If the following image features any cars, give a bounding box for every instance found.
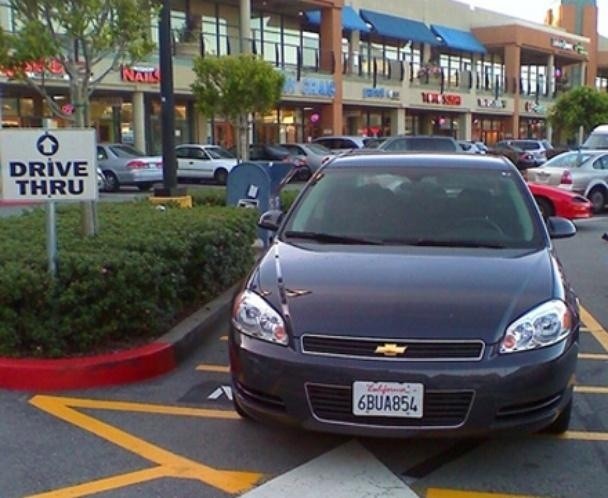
[524,124,608,216]
[314,135,490,154]
[175,144,241,183]
[226,138,336,179]
[225,149,582,442]
[95,141,163,191]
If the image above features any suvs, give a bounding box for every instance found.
[494,139,554,169]
